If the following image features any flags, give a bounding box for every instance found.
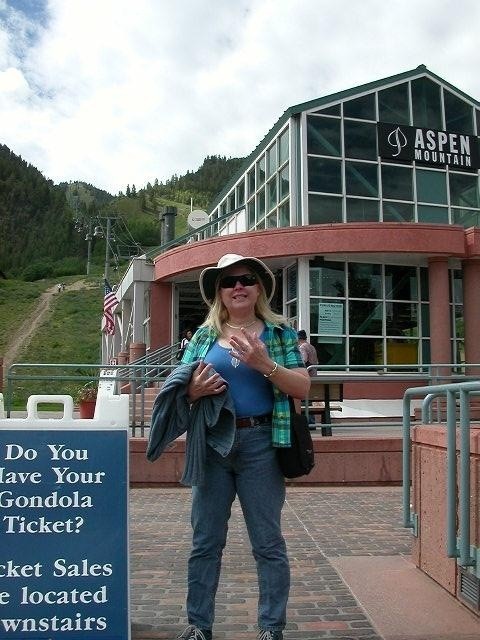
[101,278,119,335]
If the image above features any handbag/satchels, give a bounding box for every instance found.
[275,394,316,480]
[176,338,186,359]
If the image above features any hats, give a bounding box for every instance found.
[198,253,277,309]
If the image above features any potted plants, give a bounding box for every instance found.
[67,383,97,419]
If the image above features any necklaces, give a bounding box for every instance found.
[225,318,258,330]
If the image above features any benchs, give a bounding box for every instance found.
[297,405,342,437]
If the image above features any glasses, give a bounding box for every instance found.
[217,273,260,290]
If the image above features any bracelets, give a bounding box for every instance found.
[264,362,278,378]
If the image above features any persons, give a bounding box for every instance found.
[146,253,311,640]
[181,329,193,349]
[297,330,318,430]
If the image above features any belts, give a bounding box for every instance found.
[234,411,272,429]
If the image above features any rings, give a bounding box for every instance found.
[241,351,243,354]
